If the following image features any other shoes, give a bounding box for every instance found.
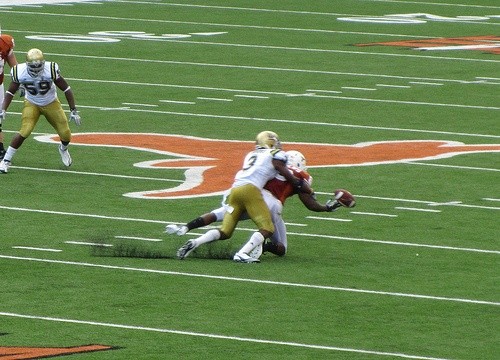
[0,150,6,159]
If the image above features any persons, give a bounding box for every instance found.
[165,149,343,260]
[177,130,313,263]
[0,48,81,173]
[0,34,19,159]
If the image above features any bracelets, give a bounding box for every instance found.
[71,108,75,110]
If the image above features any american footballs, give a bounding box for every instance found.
[335,188,357,209]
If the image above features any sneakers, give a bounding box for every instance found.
[59,144,72,167]
[0,158,11,173]
[250,238,266,259]
[176,239,198,260]
[166,225,189,236]
[233,252,261,263]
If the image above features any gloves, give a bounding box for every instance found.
[0,107,6,120]
[70,108,81,126]
[326,199,343,212]
[17,83,25,99]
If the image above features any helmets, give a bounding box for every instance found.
[255,131,280,149]
[286,150,306,170]
[26,48,45,67]
